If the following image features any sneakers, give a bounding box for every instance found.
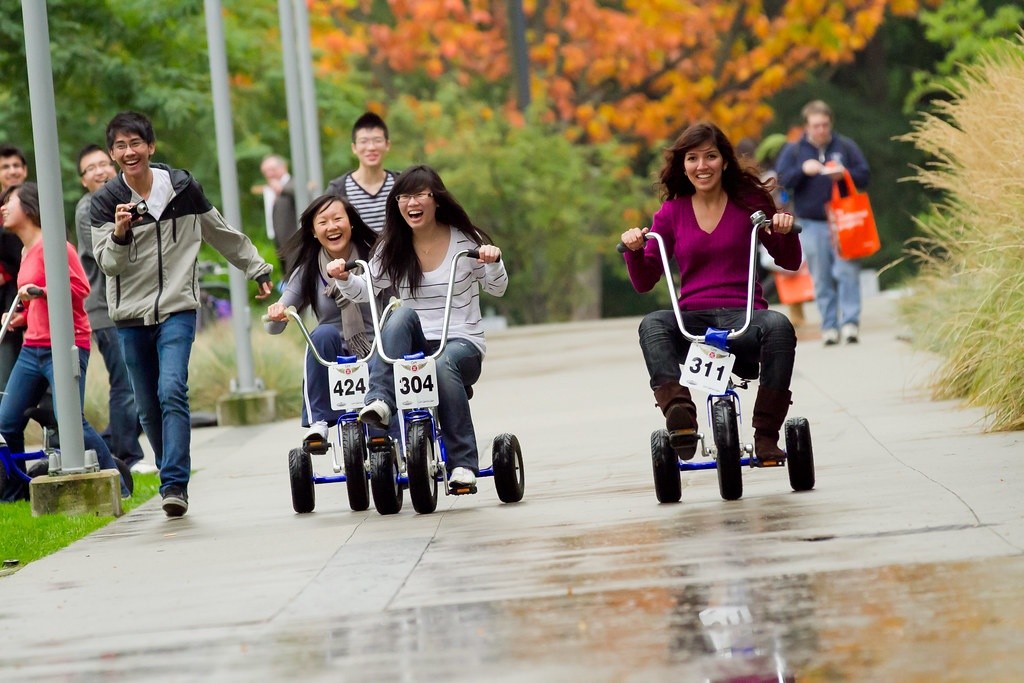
[448,466,477,489]
[162,484,188,516]
[360,400,390,431]
[303,421,329,455]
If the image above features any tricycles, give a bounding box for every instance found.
[615,208,817,502]
[0,286,137,502]
[261,297,413,513]
[327,249,525,514]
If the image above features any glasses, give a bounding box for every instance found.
[394,192,434,202]
[80,160,113,176]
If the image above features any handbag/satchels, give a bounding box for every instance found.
[823,162,881,260]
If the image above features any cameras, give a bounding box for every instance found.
[126,199,149,223]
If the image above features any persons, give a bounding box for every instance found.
[76,145,144,470]
[0,182,131,501]
[265,193,400,475]
[327,165,508,488]
[325,111,401,234]
[738,99,871,342]
[0,146,60,478]
[91,112,273,520]
[261,156,296,275]
[623,123,805,461]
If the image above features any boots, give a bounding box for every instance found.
[654,380,698,460]
[752,388,792,462]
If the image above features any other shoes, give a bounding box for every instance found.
[129,458,158,476]
[841,322,859,343]
[822,326,838,346]
